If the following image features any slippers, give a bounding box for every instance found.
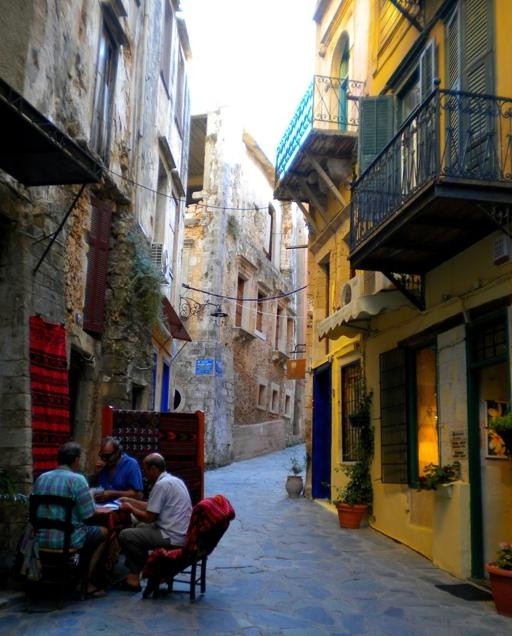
[86,588,106,597]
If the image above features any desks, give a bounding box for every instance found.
[83,498,133,562]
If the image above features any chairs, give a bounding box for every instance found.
[24,493,91,610]
[152,496,223,600]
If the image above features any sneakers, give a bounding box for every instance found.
[114,580,141,591]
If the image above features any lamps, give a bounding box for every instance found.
[179,296,228,327]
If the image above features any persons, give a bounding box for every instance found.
[31,439,192,598]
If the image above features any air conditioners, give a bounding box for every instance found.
[149,242,169,276]
[340,277,361,308]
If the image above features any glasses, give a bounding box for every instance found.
[98,451,115,458]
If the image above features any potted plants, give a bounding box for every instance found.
[286,456,307,498]
[333,377,375,529]
[485,542,511,618]
